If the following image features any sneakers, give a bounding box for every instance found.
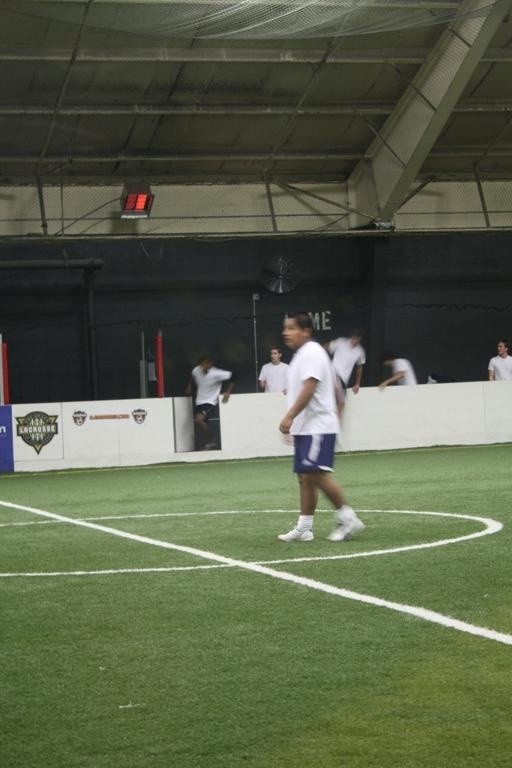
[204,442,218,449]
[327,519,366,542]
[278,527,314,543]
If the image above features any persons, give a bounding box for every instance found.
[487,337,512,381]
[278,311,365,543]
[323,327,366,393]
[257,348,288,393]
[377,350,418,390]
[186,355,234,450]
